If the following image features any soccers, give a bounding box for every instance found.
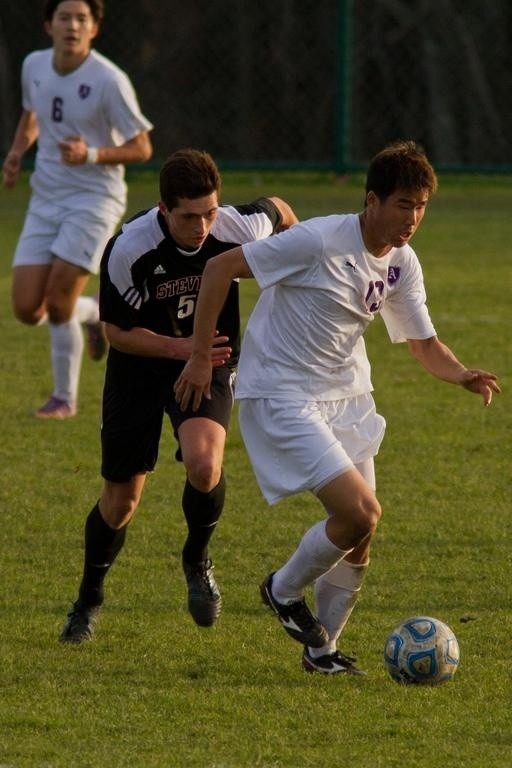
[384,617,459,684]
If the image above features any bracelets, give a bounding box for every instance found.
[83,148,98,167]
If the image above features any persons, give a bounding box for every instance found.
[173,142,502,677]
[59,147,300,644]
[3,0,156,419]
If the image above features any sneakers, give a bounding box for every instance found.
[34,396,76,418]
[260,572,364,676]
[59,603,101,642]
[183,559,221,627]
[87,295,108,360]
